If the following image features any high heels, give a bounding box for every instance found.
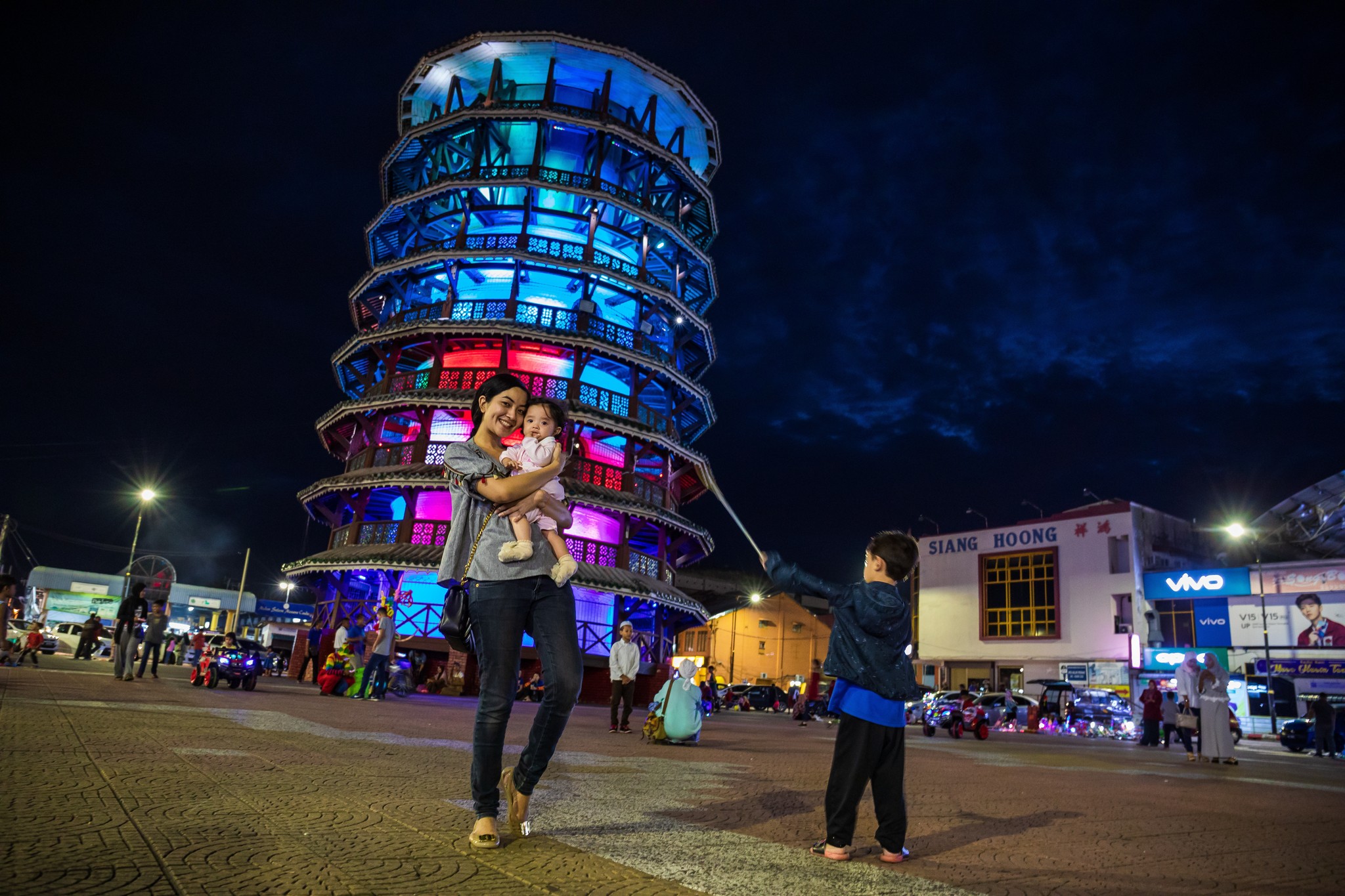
[1187,752,1195,761]
[1197,752,1208,763]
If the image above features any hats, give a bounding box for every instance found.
[620,621,632,629]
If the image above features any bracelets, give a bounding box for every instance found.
[541,496,550,510]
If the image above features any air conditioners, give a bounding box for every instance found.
[1118,623,1133,633]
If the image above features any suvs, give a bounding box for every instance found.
[1278,703,1345,753]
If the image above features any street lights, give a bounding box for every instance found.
[729,594,760,684]
[279,582,295,604]
[1226,523,1277,735]
[121,488,155,598]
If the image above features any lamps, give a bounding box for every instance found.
[1021,499,1043,519]
[918,514,939,535]
[1084,488,1101,502]
[966,508,988,529]
[577,299,596,313]
[637,320,653,335]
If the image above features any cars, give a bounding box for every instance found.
[131,628,288,673]
[716,683,787,713]
[1227,707,1242,746]
[907,690,980,721]
[921,698,989,741]
[50,622,113,659]
[3,618,60,655]
[191,645,257,692]
[971,692,1039,727]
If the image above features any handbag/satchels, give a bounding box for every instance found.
[438,585,474,654]
[641,712,667,744]
[1176,708,1197,729]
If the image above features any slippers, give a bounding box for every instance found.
[881,847,910,863]
[810,839,850,861]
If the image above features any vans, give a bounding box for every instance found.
[1025,679,1133,729]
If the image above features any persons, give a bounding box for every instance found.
[649,658,704,744]
[15,622,45,668]
[765,683,779,714]
[84,616,103,659]
[498,398,578,588]
[408,648,427,685]
[959,682,976,713]
[188,628,205,667]
[1004,690,1018,721]
[262,645,276,677]
[797,658,838,729]
[0,571,18,651]
[114,581,148,681]
[515,669,544,702]
[317,606,398,701]
[1174,650,1210,762]
[1295,593,1345,646]
[296,619,324,685]
[1300,692,1337,758]
[439,661,465,696]
[134,599,168,679]
[219,632,237,656]
[72,613,100,660]
[792,694,816,722]
[175,632,190,666]
[762,530,919,861]
[159,629,177,665]
[426,665,448,694]
[274,651,284,677]
[1136,679,1189,750]
[609,621,640,733]
[1195,652,1239,766]
[699,665,751,717]
[435,372,582,849]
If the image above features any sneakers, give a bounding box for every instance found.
[349,693,365,701]
[366,693,381,702]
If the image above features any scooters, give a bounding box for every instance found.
[387,659,414,699]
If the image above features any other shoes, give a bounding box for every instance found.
[1223,758,1239,766]
[798,722,807,727]
[124,674,134,680]
[502,766,530,838]
[116,676,122,681]
[620,725,632,733]
[469,818,500,848]
[1212,757,1219,764]
[609,725,618,733]
